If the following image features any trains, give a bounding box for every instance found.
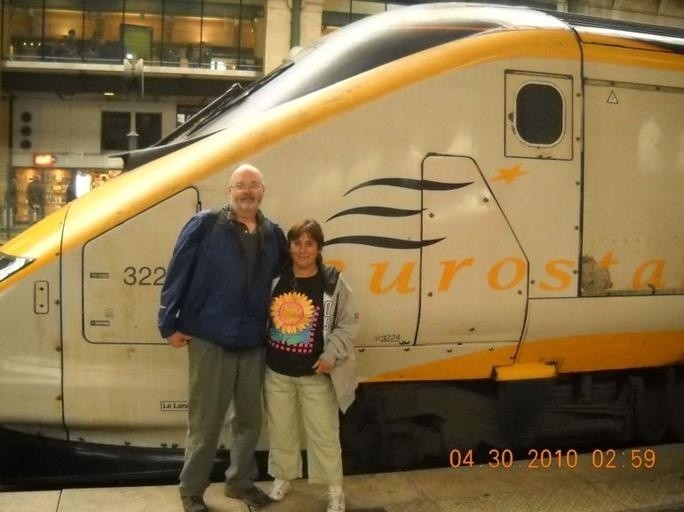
[0,1,684,464]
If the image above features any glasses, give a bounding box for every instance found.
[229,182,262,191]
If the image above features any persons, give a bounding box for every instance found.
[5,175,45,226]
[167,41,212,69]
[64,29,107,58]
[158,163,289,512]
[262,219,359,512]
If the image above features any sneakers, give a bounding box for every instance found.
[180,495,209,512]
[267,477,293,503]
[326,484,346,512]
[224,487,272,508]
